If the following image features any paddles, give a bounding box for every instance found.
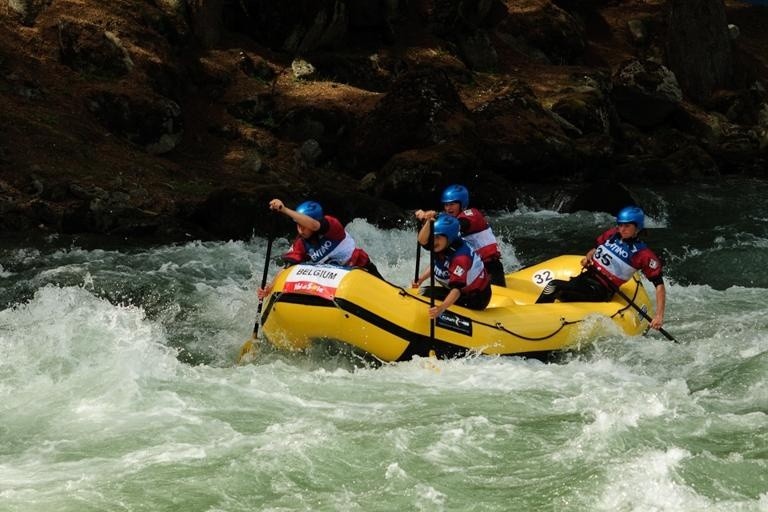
[234,201,282,366]
[419,213,444,375]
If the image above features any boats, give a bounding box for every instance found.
[260,253,654,365]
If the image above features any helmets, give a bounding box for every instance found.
[615,205,645,232]
[432,212,463,246]
[441,183,470,211]
[295,200,324,221]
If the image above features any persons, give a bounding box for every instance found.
[258,199,384,300]
[417,211,491,320]
[411,185,507,289]
[535,207,665,329]
